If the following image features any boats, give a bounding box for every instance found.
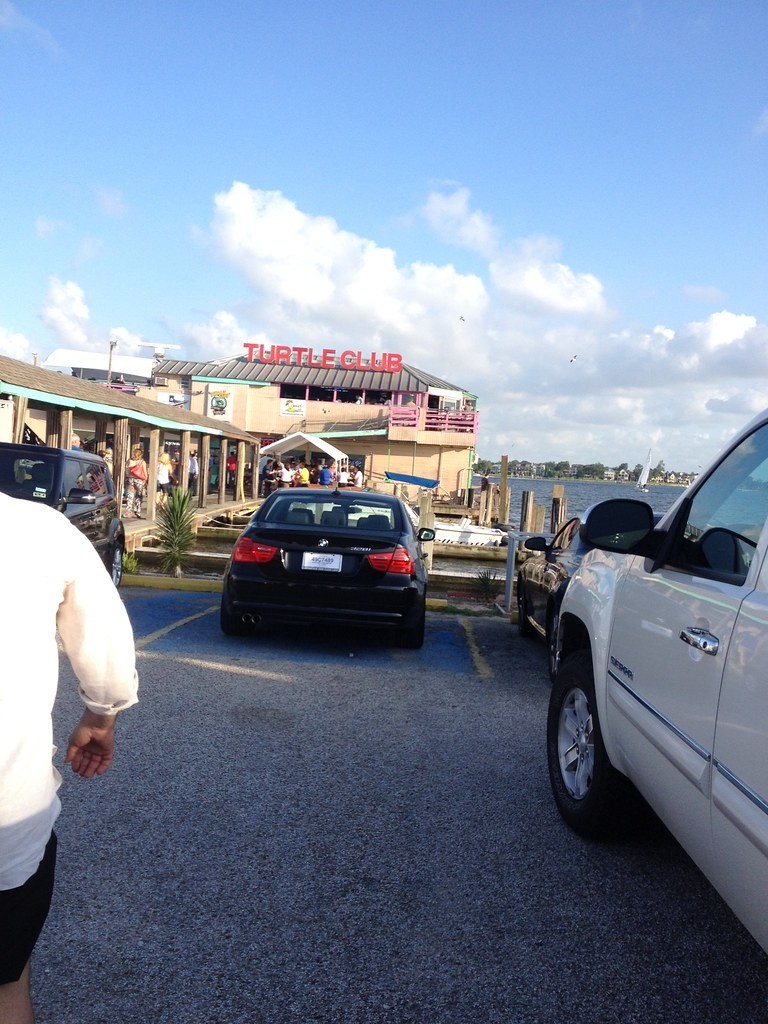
[384,472,514,547]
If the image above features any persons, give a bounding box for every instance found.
[262,459,363,489]
[112,377,125,384]
[482,473,490,491]
[72,433,86,451]
[157,452,178,506]
[464,401,474,410]
[384,396,393,405]
[354,394,365,405]
[96,449,114,478]
[244,464,252,485]
[226,452,237,488]
[0,490,140,1023]
[122,448,147,518]
[407,398,416,405]
[188,454,199,496]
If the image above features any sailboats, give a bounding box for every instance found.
[635,448,652,493]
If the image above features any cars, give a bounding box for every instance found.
[515,512,672,681]
[220,487,436,652]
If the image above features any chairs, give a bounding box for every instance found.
[21,464,53,492]
[285,509,390,532]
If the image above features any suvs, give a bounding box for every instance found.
[0,441,127,591]
[540,405,768,958]
